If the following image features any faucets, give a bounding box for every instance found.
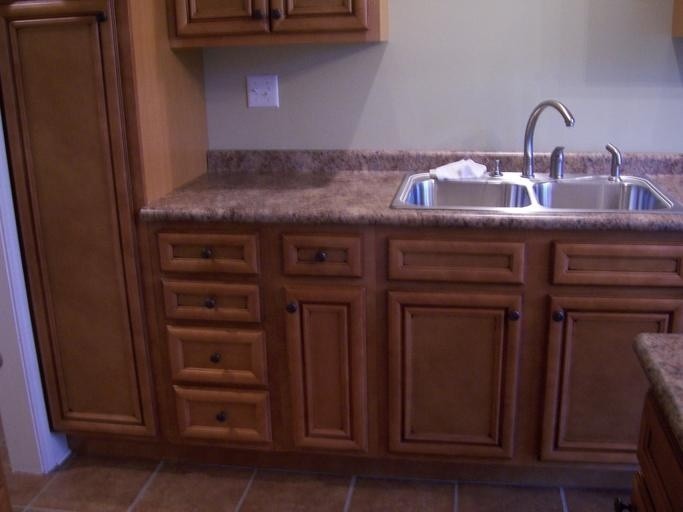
[523,100,575,177]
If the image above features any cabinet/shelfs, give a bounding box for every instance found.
[0,2,208,463]
[541,227,683,489]
[383,223,528,492]
[164,1,389,49]
[139,223,278,491]
[282,224,372,491]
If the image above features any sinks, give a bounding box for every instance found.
[533,175,683,214]
[390,172,533,215]
